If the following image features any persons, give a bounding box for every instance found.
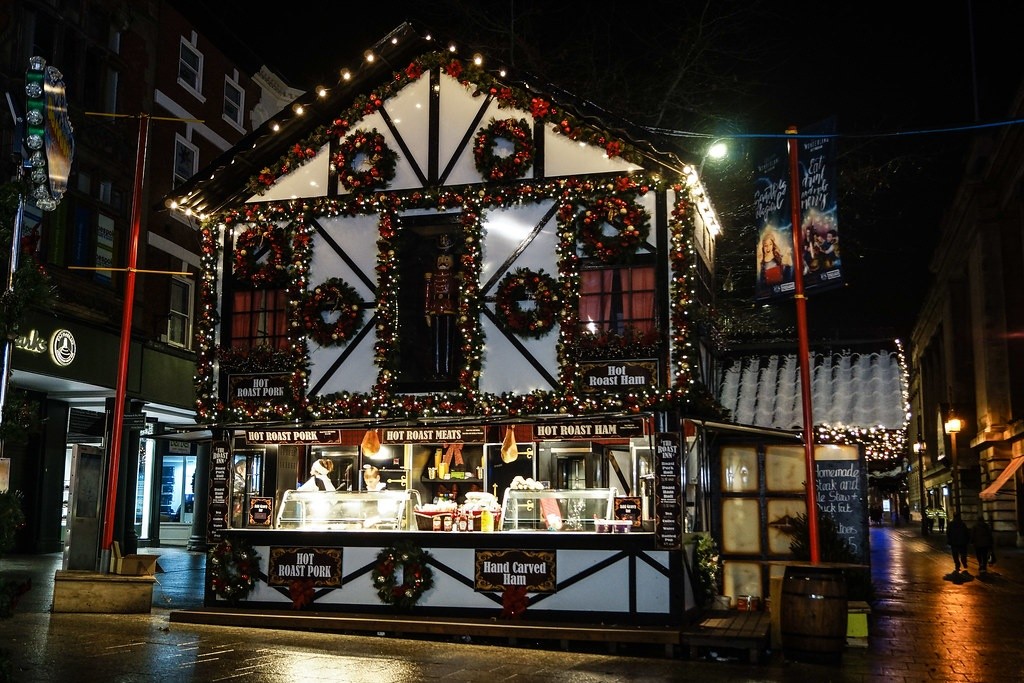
[760,234,793,287]
[425,233,460,381]
[869,504,883,524]
[973,514,993,571]
[800,208,840,273]
[948,513,969,570]
[363,466,388,494]
[233,460,246,493]
[297,459,337,492]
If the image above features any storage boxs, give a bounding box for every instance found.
[113,541,165,576]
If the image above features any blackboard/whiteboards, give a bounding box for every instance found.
[812,457,871,599]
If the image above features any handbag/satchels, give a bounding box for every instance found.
[987,552,996,564]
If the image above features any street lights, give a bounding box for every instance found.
[913,433,930,537]
[944,418,962,519]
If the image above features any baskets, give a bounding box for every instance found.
[413,510,501,531]
